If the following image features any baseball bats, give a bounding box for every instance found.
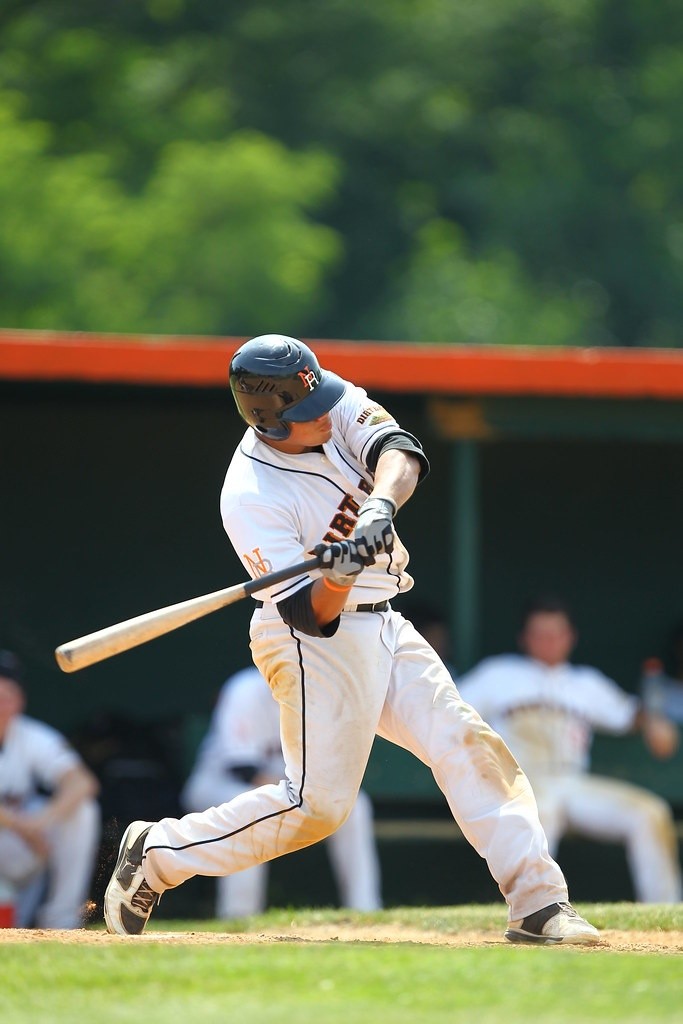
[53,557,321,677]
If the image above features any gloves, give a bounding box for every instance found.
[314,539,365,591]
[350,495,398,567]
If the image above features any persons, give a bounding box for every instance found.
[1,667,100,928]
[105,334,600,944]
[186,667,382,917]
[456,609,681,902]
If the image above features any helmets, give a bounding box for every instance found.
[228,334,346,443]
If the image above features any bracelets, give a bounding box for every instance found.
[324,577,352,592]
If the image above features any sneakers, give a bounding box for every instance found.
[504,900,599,946]
[103,820,160,936]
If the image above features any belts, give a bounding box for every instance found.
[255,600,391,614]
